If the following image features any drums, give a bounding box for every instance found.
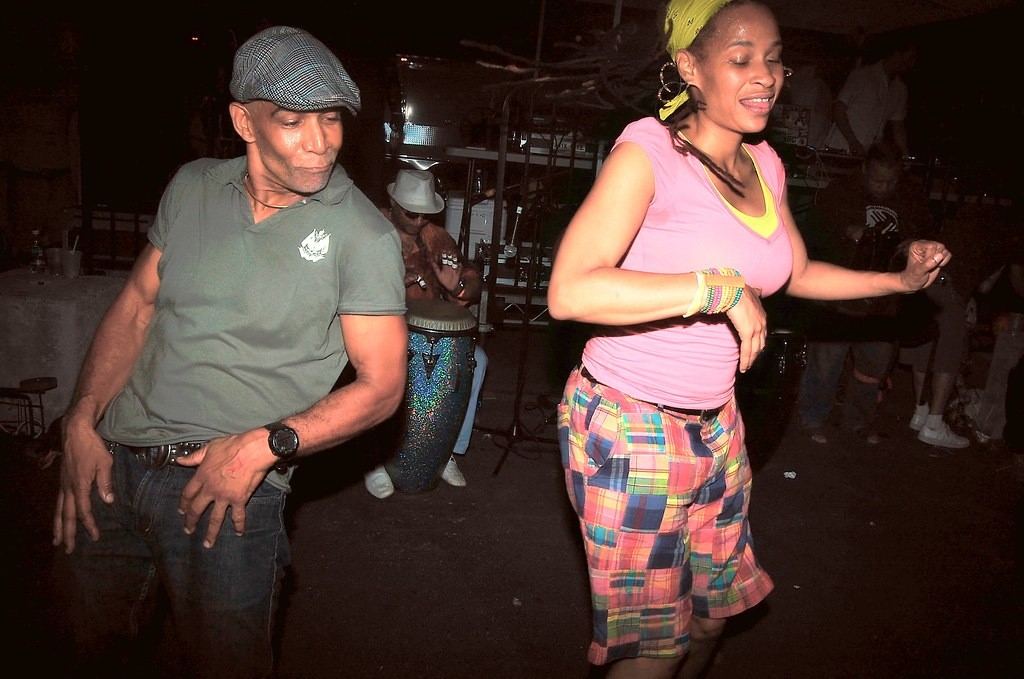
[379,297,479,494]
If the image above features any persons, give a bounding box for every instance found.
[800,143,1024,448]
[51,27,407,679]
[547,0,953,679]
[825,35,917,161]
[365,169,489,498]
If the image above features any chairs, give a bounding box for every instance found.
[815,236,942,444]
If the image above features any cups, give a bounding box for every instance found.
[45,248,67,276]
[62,250,82,278]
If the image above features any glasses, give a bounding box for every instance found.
[402,208,430,219]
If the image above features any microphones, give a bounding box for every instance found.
[504,200,525,257]
[542,80,596,103]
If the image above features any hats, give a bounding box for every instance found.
[387,168,445,213]
[230,26,361,117]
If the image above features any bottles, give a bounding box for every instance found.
[29,230,45,280]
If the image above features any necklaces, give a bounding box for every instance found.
[245,173,287,208]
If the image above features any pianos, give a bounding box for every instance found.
[805,145,941,173]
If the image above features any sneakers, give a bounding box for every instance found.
[917,422,970,449]
[909,414,930,431]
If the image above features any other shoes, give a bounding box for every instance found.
[808,429,833,448]
[361,461,393,498]
[442,456,467,488]
[855,429,881,446]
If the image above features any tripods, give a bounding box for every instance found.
[473,102,559,478]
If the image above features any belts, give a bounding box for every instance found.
[131,441,209,470]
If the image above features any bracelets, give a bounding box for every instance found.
[450,280,464,298]
[683,267,746,318]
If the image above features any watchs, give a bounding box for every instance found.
[263,421,299,470]
